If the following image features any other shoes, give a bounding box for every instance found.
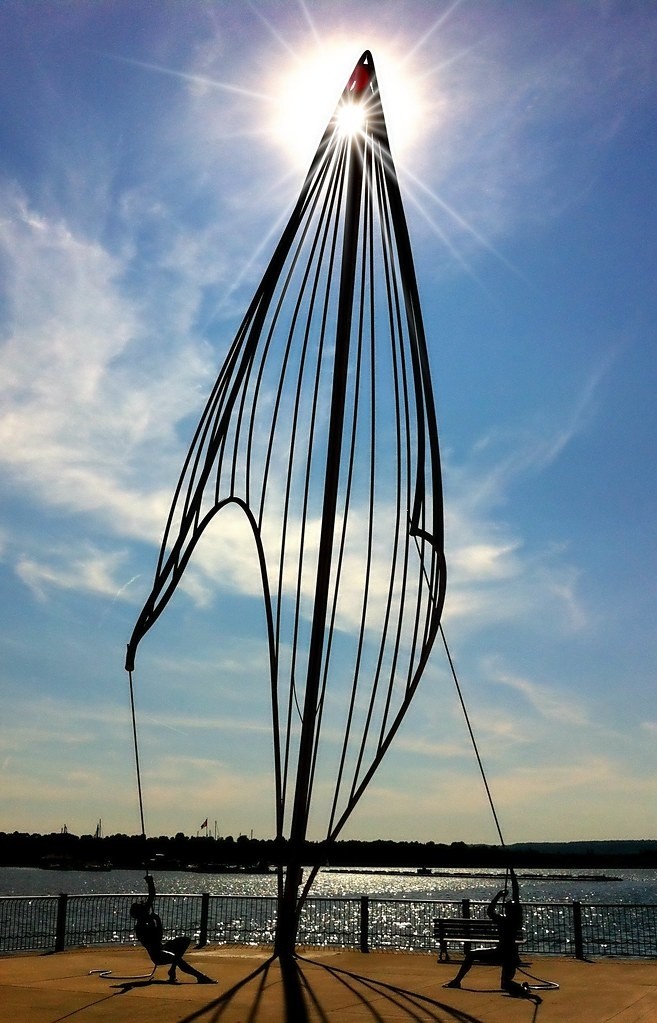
[197,975,219,984]
[168,971,178,981]
[442,980,461,988]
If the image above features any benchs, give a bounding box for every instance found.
[434,918,528,963]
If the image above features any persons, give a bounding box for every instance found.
[443,863,530,993]
[130,874,220,984]
